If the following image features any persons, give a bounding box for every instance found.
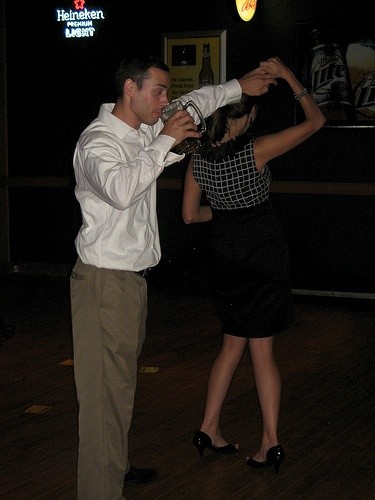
[182,57,326,467]
[70,54,276,500]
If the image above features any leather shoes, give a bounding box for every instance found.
[123,466,159,485]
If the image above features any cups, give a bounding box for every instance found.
[157,101,207,155]
[344,43,375,121]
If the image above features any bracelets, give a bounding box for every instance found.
[294,88,308,100]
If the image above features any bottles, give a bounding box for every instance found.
[198,42,214,89]
[307,26,356,125]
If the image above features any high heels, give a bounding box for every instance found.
[192,428,239,463]
[246,444,286,474]
